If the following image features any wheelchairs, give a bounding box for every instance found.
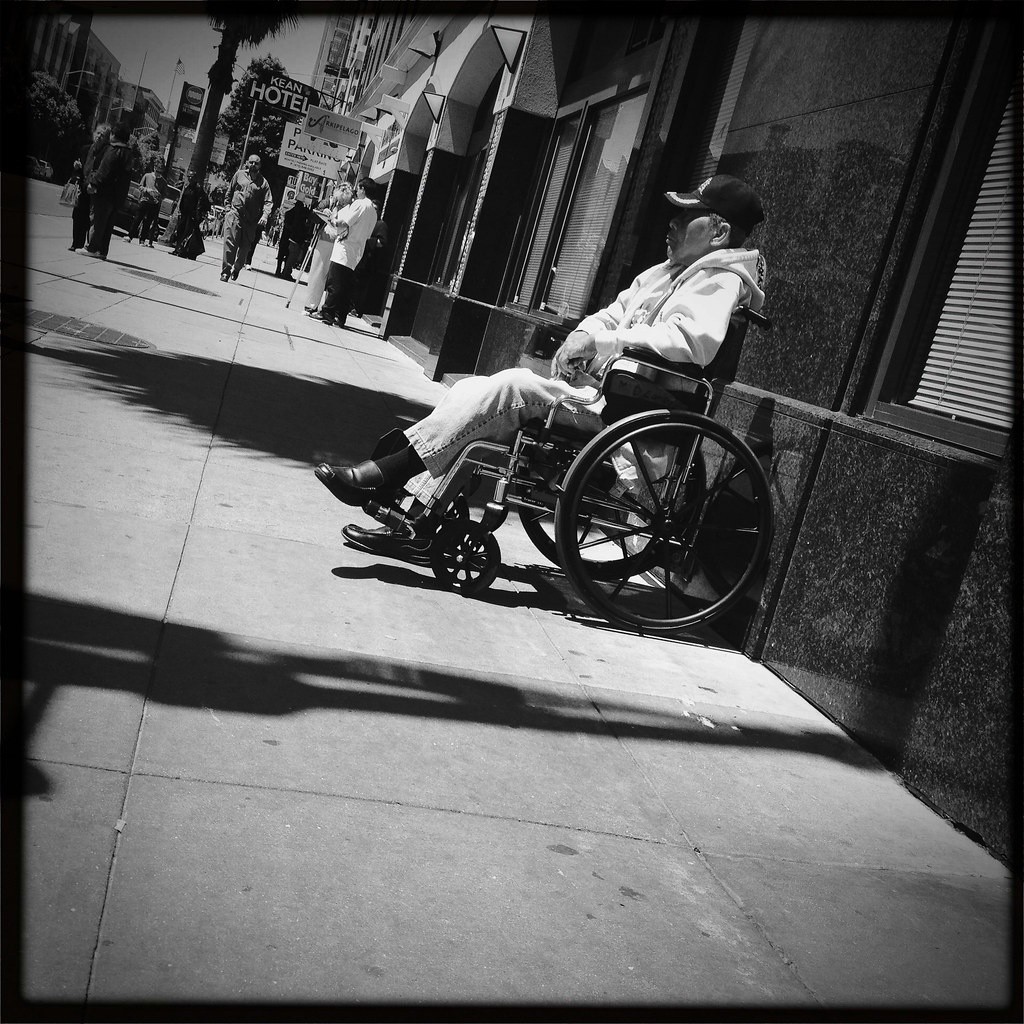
[357,297,780,639]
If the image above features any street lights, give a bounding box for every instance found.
[132,126,155,137]
[59,71,95,93]
[106,107,133,124]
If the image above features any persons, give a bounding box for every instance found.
[67,122,133,261]
[300,177,389,329]
[266,226,280,246]
[219,154,273,280]
[124,158,167,249]
[167,170,209,258]
[312,173,766,570]
[274,200,323,282]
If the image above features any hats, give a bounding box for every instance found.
[663,174,765,237]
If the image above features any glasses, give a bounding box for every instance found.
[155,170,162,174]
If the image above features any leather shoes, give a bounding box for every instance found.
[316,459,391,506]
[340,524,433,567]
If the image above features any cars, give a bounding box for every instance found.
[113,181,160,243]
[154,184,183,235]
[27,155,54,180]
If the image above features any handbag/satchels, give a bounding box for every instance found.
[58,169,82,208]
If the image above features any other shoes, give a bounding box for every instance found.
[68,247,78,251]
[220,273,229,281]
[332,321,343,328]
[139,242,145,247]
[303,306,317,317]
[76,248,106,259]
[168,250,187,258]
[308,312,331,327]
[231,271,239,280]
[275,272,293,281]
[244,264,251,271]
[122,236,131,243]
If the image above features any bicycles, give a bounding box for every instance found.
[203,205,224,241]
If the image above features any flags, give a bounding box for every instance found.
[176,59,185,77]
